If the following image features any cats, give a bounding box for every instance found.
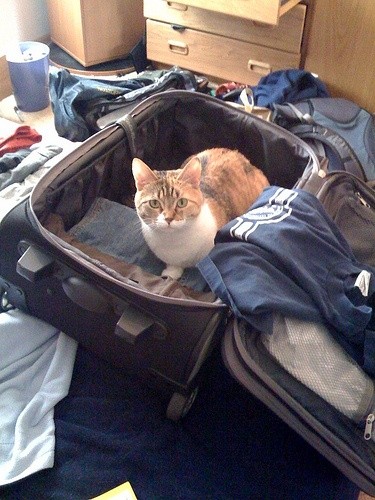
[130,147,271,280]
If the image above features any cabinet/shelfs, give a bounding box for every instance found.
[46,0,146,67]
[142,0,375,114]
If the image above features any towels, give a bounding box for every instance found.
[0,308,77,486]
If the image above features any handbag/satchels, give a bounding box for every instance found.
[225,88,273,123]
[48,65,197,142]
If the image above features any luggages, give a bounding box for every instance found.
[0,90,375,499]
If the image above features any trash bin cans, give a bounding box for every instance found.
[5,40,51,112]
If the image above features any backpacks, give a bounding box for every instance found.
[272,97,375,190]
